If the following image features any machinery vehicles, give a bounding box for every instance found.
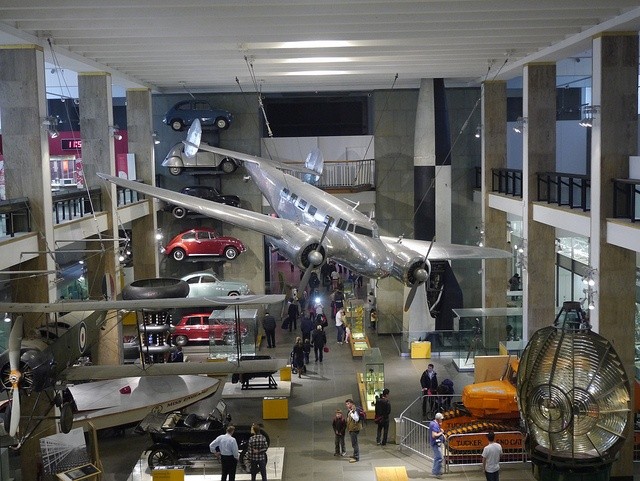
[439,354,591,461]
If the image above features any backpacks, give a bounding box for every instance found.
[356,407,366,423]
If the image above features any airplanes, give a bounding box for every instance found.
[0,272,290,449]
[95,118,512,311]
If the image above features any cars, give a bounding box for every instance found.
[173,314,248,345]
[163,187,241,220]
[181,272,249,297]
[163,141,241,176]
[165,228,245,261]
[162,100,232,131]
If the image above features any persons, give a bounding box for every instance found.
[344,322,352,345]
[301,260,374,324]
[287,299,300,332]
[428,412,447,480]
[332,410,346,458]
[247,425,269,481]
[335,307,346,345]
[303,339,312,365]
[420,364,438,424]
[370,309,376,331]
[261,312,277,349]
[509,274,521,302]
[373,389,391,446]
[481,431,504,481]
[292,337,307,379]
[345,399,364,464]
[435,379,456,415]
[299,314,313,343]
[208,426,240,481]
[311,325,327,364]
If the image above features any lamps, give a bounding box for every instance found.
[580,267,596,286]
[118,245,130,263]
[577,103,598,129]
[515,244,527,253]
[511,115,527,133]
[43,115,59,138]
[151,130,161,145]
[78,257,85,266]
[112,123,123,141]
[474,125,483,139]
[155,227,163,242]
[581,288,597,308]
[3,311,11,323]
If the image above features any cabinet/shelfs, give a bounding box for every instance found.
[341,279,370,357]
[207,308,258,376]
[357,348,384,420]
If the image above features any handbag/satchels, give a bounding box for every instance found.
[323,347,329,353]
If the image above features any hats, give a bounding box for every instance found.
[435,413,444,419]
